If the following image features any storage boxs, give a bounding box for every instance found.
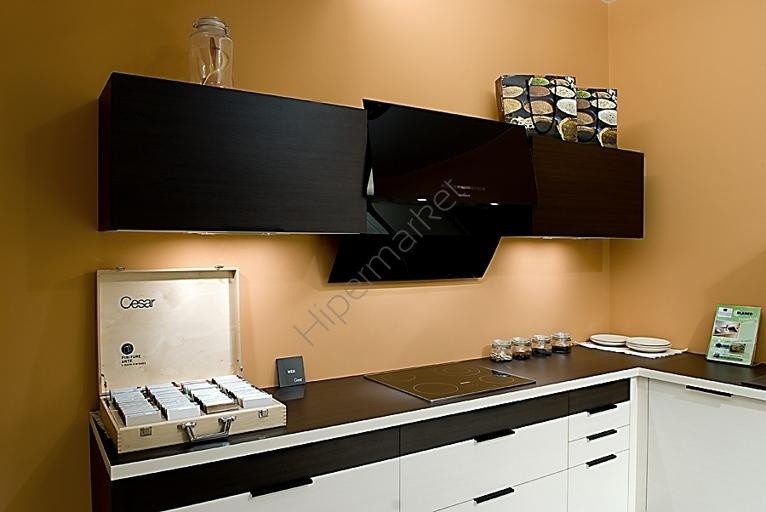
[91,263,288,454]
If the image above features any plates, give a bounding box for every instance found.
[589,334,628,346]
[625,337,672,353]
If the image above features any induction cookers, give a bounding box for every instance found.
[365,362,535,403]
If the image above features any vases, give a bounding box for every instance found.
[188,11,237,90]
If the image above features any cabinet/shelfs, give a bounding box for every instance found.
[83,339,642,512]
[633,350,766,512]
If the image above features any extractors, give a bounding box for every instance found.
[328,99,539,282]
